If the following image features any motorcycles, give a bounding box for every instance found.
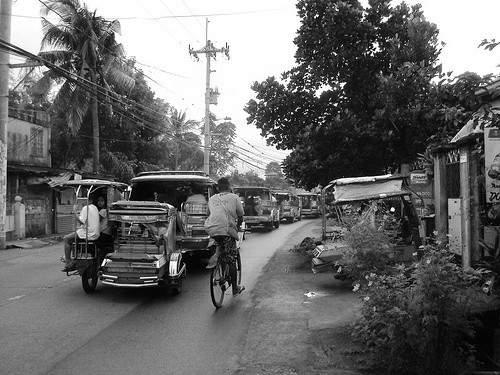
[65,179,128,294]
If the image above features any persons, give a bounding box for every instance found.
[60,193,101,272]
[203,177,245,297]
[60,195,114,263]
[176,183,208,237]
[254,191,268,216]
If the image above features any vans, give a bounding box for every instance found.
[297,193,323,217]
[227,187,279,232]
[273,193,301,223]
[131,171,245,266]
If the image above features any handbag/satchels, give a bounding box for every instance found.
[228,221,239,240]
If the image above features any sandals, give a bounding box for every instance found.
[233,286,245,297]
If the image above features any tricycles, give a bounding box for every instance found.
[98,200,186,295]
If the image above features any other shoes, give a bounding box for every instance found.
[61,266,71,272]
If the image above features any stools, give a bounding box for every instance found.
[73,241,97,258]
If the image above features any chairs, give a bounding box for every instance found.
[185,194,207,234]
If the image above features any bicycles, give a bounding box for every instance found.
[209,228,251,308]
